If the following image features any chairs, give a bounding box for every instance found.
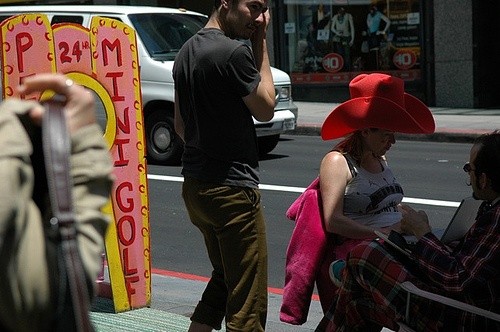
[397,281,500,332]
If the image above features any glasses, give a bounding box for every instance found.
[464,163,480,174]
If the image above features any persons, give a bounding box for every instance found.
[172,0,275,332]
[330,8,355,72]
[366,6,391,69]
[315,72,436,315]
[313,128,500,332]
[0,73,117,332]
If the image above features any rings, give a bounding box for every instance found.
[59,77,74,96]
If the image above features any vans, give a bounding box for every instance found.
[0,4,299,166]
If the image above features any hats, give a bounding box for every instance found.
[320,73,435,139]
